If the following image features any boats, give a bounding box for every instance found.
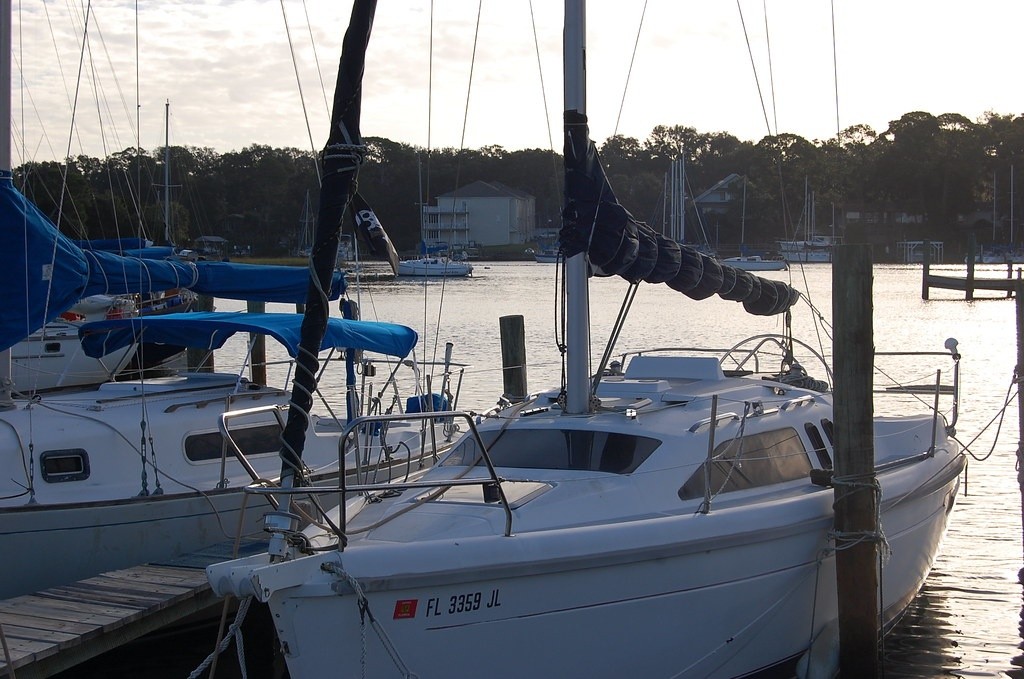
[0,0,974,679]
